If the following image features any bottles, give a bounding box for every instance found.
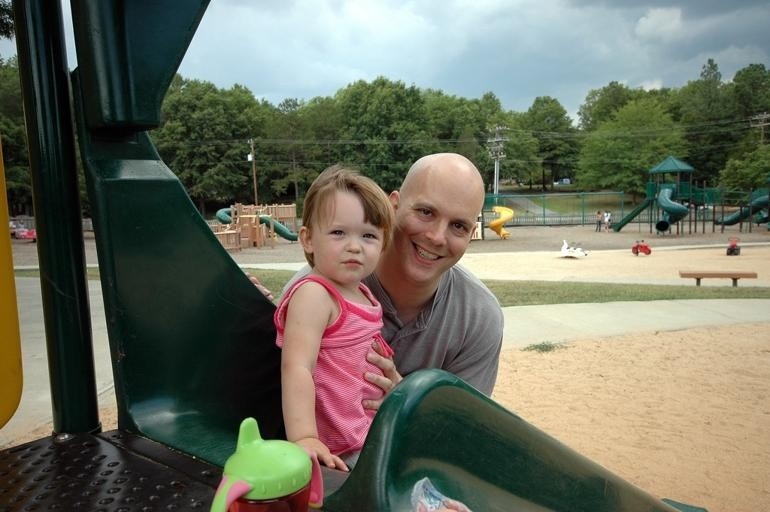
[210,416,325,512]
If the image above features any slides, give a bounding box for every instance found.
[655,188,688,231]
[715,196,770,225]
[79,124,706,512]
[490,206,514,240]
[607,182,655,232]
[217,209,298,241]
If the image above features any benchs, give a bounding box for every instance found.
[679,268,759,287]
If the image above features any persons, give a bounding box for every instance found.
[416,495,471,512]
[604,211,612,230]
[272,164,397,475]
[595,211,602,232]
[244,152,502,419]
